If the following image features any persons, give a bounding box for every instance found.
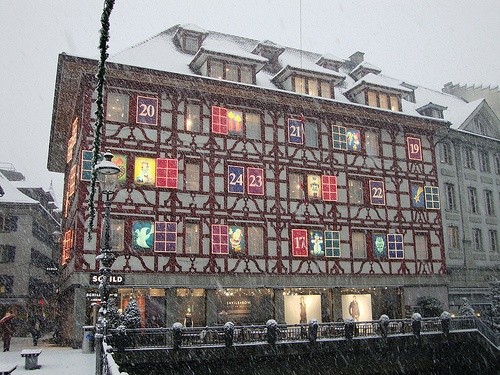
[184,308,193,327]
[52,315,62,338]
[298,297,307,324]
[2,319,14,352]
[29,319,48,346]
[349,295,360,321]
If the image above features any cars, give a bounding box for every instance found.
[465,302,495,322]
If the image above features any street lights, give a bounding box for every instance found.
[91,149,122,375]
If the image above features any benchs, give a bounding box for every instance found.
[21,349,43,370]
[0,362,19,375]
